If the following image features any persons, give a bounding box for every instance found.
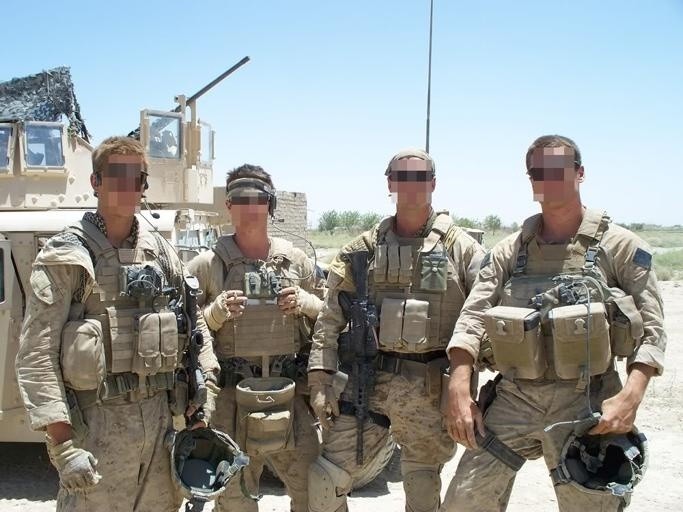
[14,137,666,512]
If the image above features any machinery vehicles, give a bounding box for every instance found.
[0,56,251,444]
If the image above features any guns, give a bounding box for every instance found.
[473,374,527,471]
[336,251,379,466]
[178,274,205,431]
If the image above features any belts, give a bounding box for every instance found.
[376,354,448,377]
[213,368,263,387]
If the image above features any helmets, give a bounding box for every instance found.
[164,427,250,504]
[560,413,650,496]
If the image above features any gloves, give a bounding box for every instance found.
[306,370,340,432]
[276,286,324,320]
[49,439,104,493]
[202,290,247,333]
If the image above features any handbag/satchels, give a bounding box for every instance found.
[234,374,297,450]
[58,302,193,393]
[483,289,646,382]
[377,294,430,355]
